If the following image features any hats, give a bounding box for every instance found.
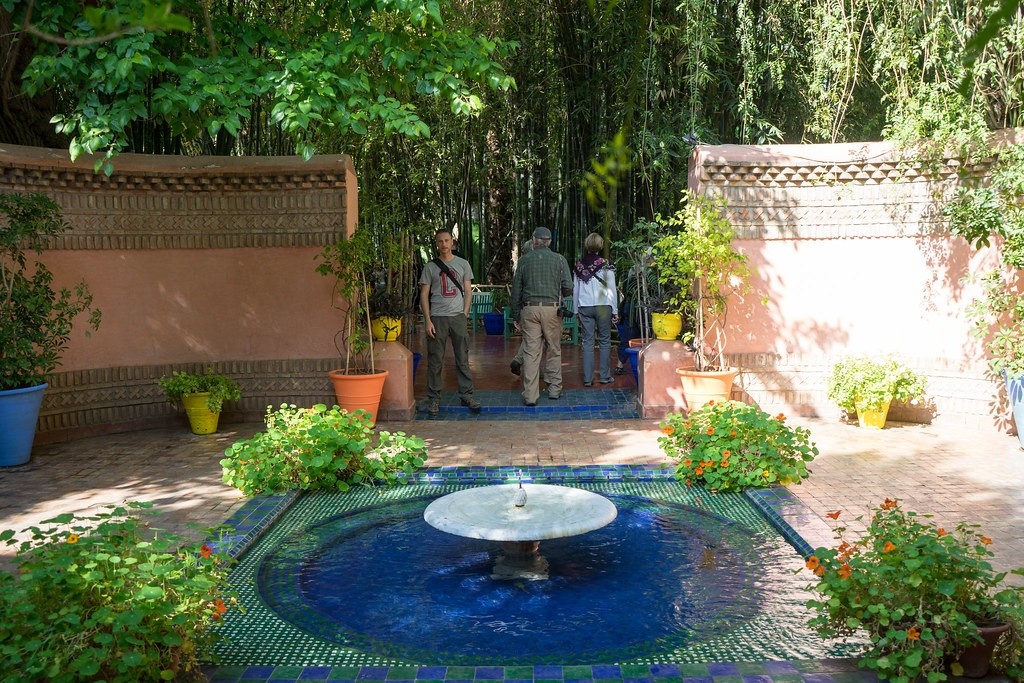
[533,227,552,240]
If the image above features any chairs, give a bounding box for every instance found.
[502,297,580,346]
[462,292,496,333]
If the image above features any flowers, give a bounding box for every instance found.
[794,497,1024,683]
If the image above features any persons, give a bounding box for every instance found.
[574,232,618,386]
[510,239,545,379]
[418,228,481,414]
[615,246,654,374]
[511,227,574,405]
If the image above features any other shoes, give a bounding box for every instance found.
[615,366,624,375]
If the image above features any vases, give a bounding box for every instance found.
[954,621,1011,678]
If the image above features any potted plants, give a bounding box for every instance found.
[942,149,1024,450]
[608,189,770,410]
[828,353,939,430]
[312,221,414,429]
[159,368,241,435]
[0,191,106,466]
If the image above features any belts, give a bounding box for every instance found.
[524,302,561,306]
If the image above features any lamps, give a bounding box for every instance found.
[681,130,700,146]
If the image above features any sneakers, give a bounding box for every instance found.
[583,380,593,386]
[461,398,481,410]
[525,397,536,406]
[509,359,521,376]
[430,399,439,413]
[549,390,564,398]
[599,377,614,384]
